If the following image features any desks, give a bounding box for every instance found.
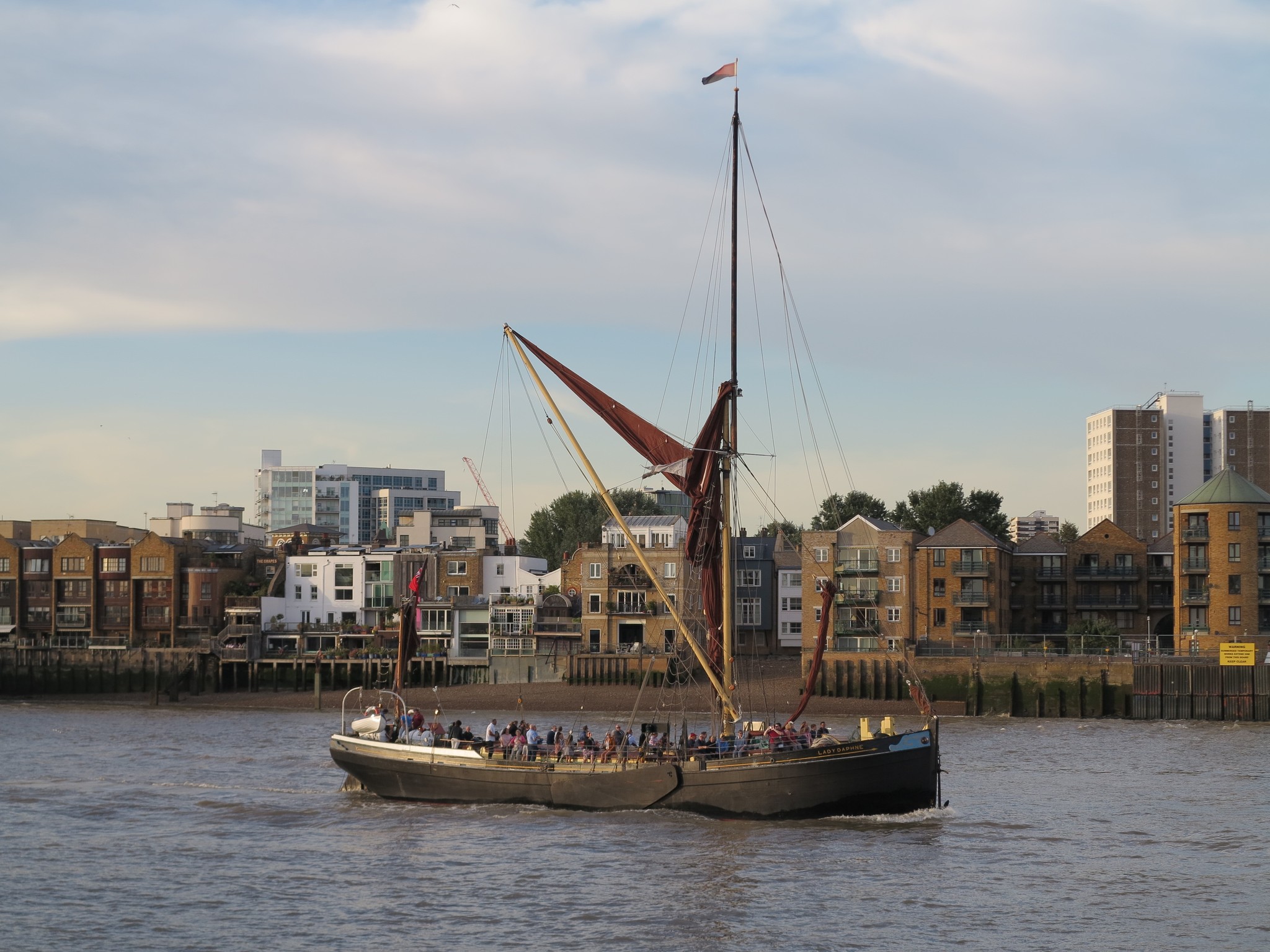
[480,630,486,634]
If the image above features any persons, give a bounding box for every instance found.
[380,708,393,743]
[463,726,473,744]
[448,720,463,749]
[732,730,747,758]
[500,720,544,762]
[485,719,498,759]
[687,731,728,761]
[580,725,596,764]
[398,708,412,740]
[546,725,574,763]
[600,725,638,764]
[763,721,829,753]
[226,642,246,649]
[639,729,674,763]
[411,708,425,730]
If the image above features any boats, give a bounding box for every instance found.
[327,688,945,822]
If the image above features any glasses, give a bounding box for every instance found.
[588,733,590,735]
[616,727,621,729]
[775,726,779,727]
[739,733,743,734]
[606,734,610,736]
[554,728,557,729]
[700,736,704,738]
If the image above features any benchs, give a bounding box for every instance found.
[461,739,691,762]
[468,627,478,634]
[746,731,789,753]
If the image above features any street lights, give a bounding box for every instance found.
[1195,629,1199,657]
[977,629,981,655]
[1147,616,1150,657]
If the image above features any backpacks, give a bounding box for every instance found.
[626,735,632,745]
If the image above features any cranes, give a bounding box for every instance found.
[463,455,516,556]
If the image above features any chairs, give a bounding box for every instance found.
[615,645,630,654]
[623,604,630,612]
[635,604,642,612]
[630,642,640,652]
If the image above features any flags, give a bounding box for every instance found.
[409,566,422,605]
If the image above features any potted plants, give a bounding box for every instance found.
[644,600,655,613]
[636,569,654,586]
[523,616,534,635]
[605,601,619,613]
[324,637,448,660]
[270,616,276,630]
[497,587,534,605]
[277,614,286,632]
[296,619,401,634]
[209,552,220,567]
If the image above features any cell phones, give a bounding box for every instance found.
[769,726,772,729]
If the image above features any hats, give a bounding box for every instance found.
[788,721,795,724]
[413,708,419,711]
[690,733,698,737]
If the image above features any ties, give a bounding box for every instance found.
[620,731,624,735]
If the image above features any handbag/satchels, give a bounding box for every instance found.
[563,747,566,750]
[512,747,519,751]
[773,737,782,745]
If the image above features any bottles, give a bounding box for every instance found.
[779,735,782,742]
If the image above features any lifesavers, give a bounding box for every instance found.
[364,706,379,716]
